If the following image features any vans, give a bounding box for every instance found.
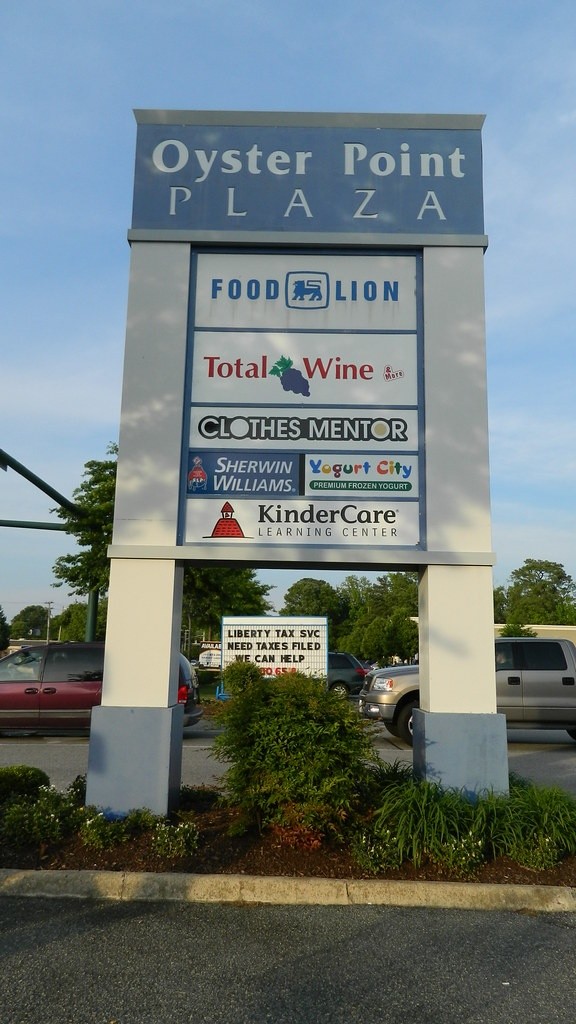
[0,640,203,732]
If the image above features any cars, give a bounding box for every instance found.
[359,635,576,741]
[326,651,367,699]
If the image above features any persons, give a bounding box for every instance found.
[45,655,71,680]
[412,656,416,665]
[496,649,512,669]
[8,651,42,679]
